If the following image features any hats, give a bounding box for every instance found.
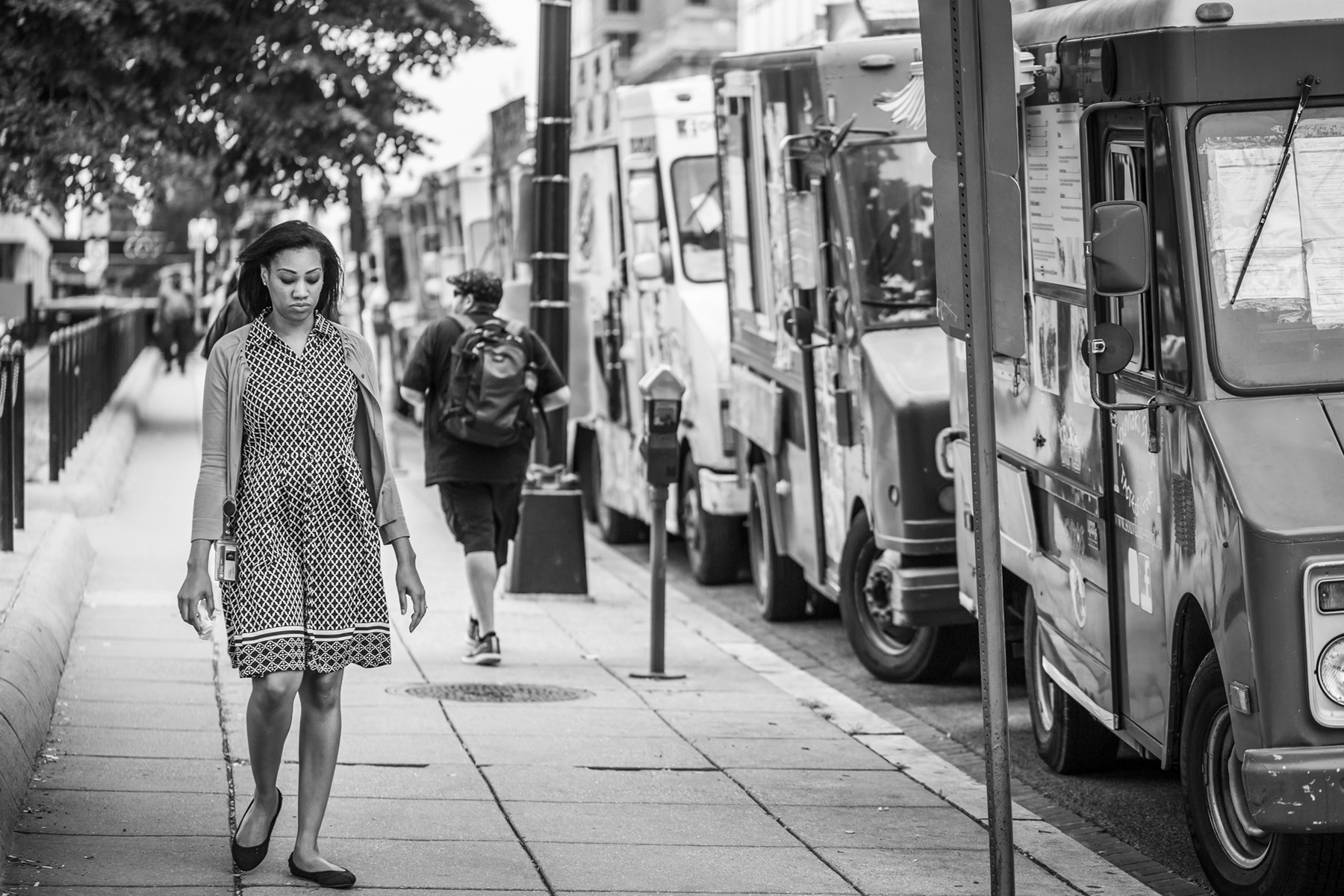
[446,268,503,302]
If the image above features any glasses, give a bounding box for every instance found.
[453,290,465,298]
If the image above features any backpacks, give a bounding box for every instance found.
[433,314,529,449]
[160,292,192,325]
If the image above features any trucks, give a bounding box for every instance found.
[938,1,1344,896]
[345,0,1025,687]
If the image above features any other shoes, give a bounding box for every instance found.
[468,614,481,643]
[231,786,284,871]
[288,848,357,890]
[461,635,502,666]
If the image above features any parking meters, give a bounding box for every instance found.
[637,364,686,676]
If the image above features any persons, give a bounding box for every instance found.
[398,267,571,665]
[153,271,252,375]
[177,220,427,888]
